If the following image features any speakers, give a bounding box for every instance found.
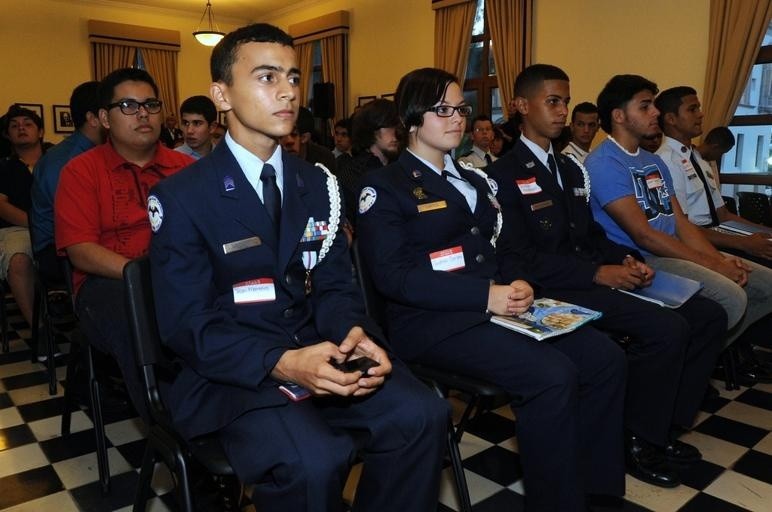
[313,83,335,118]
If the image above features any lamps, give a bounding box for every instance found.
[192,1,226,46]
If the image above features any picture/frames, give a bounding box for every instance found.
[53,104,76,132]
[15,102,43,129]
[358,96,376,107]
[219,111,228,126]
[382,93,395,102]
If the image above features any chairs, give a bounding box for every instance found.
[0,192,772,512]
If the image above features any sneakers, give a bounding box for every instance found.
[31,323,64,362]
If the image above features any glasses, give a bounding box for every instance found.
[424,103,474,118]
[111,98,165,117]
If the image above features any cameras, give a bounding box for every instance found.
[332,356,380,376]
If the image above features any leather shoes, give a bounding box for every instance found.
[625,432,684,490]
[701,343,772,402]
[655,438,706,463]
[62,373,130,412]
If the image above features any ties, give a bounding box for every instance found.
[687,152,721,227]
[259,164,283,229]
[547,151,559,185]
